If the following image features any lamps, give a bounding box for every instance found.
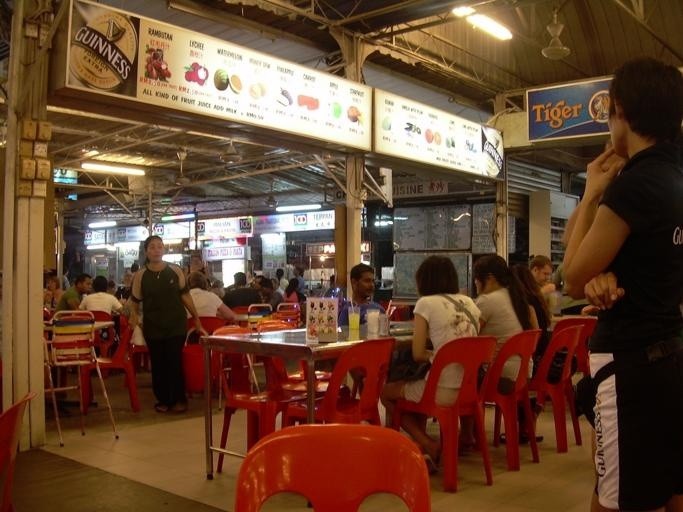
[265,180,278,208]
[541,8,571,60]
[174,152,189,186]
[220,140,242,163]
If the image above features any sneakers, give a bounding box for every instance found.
[423,454,440,476]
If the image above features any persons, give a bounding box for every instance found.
[43,255,335,373]
[131,235,202,414]
[559,140,628,246]
[460,254,533,451]
[380,257,483,472]
[338,264,387,327]
[560,57,683,512]
[499,254,592,444]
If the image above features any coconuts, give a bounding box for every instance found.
[214,69,243,93]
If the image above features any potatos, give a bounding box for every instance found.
[348,107,359,121]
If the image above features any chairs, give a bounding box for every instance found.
[0,302,599,512]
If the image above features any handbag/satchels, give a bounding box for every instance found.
[575,336,683,418]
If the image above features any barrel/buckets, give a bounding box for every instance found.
[182,327,220,392]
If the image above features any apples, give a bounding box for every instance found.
[434,132,441,143]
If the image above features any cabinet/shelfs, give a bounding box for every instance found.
[529,190,580,265]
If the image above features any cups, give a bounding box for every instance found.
[348,305,360,330]
[549,292,561,307]
[308,301,335,337]
[365,309,379,334]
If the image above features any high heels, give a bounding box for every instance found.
[463,433,477,457]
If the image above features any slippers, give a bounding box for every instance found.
[154,402,172,411]
[173,401,187,412]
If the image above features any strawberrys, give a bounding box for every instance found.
[186,63,208,85]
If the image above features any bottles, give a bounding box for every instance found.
[377,314,390,336]
[51,292,57,308]
